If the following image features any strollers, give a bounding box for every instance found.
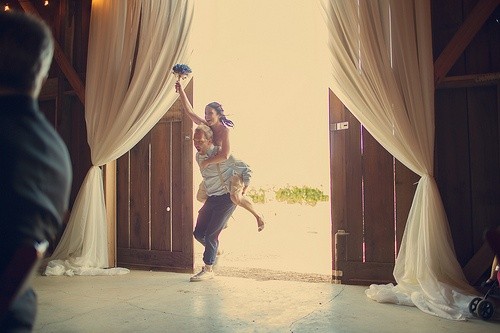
[468,220,500,321]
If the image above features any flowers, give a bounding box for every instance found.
[173,64,192,92]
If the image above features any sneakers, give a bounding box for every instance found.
[480,276,495,288]
[213,248,225,267]
[190,266,214,282]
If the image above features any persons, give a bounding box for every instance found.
[0,10,72,333]
[174,80,266,232]
[190,125,252,282]
[481,255,500,285]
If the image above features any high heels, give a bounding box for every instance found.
[257,214,267,232]
[223,215,235,229]
